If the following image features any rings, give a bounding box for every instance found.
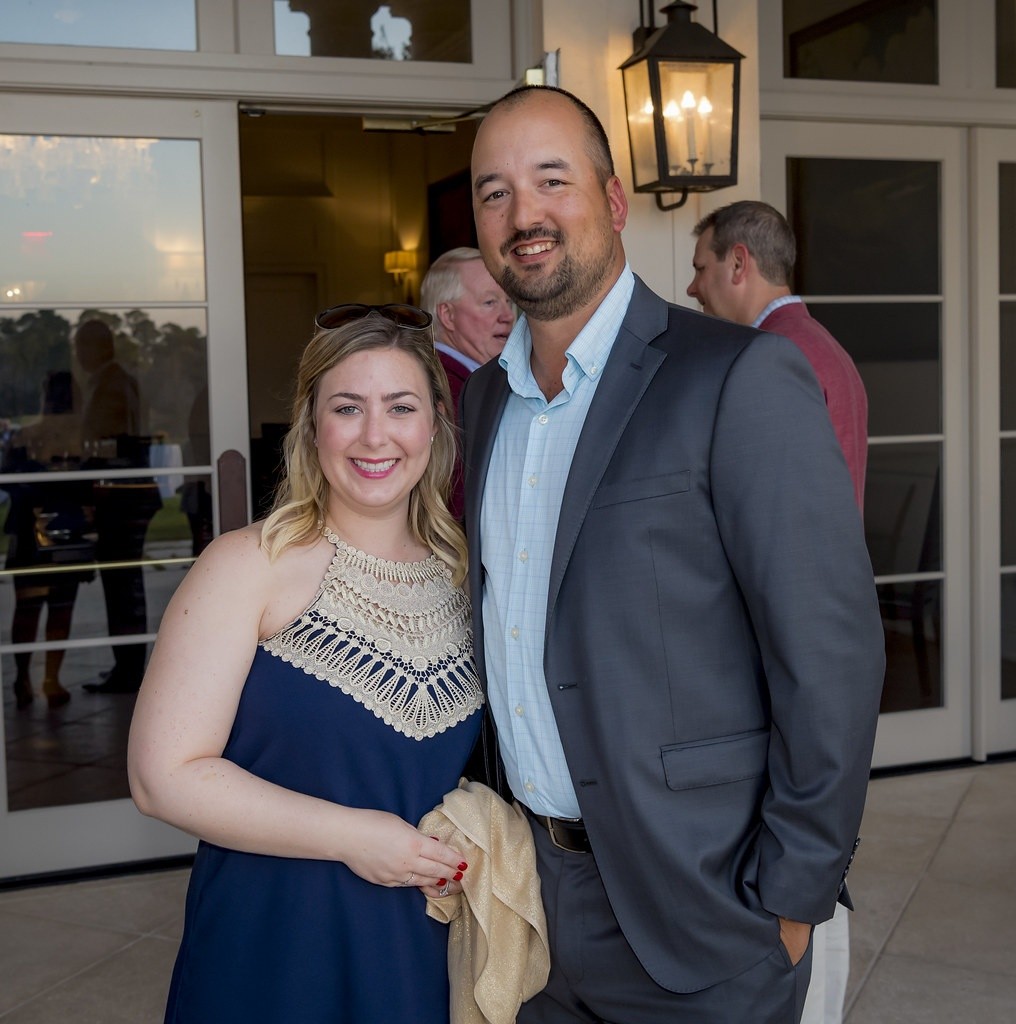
[401,872,416,885]
[438,880,451,897]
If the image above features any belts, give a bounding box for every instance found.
[514,797,594,852]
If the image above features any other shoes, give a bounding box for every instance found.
[85,670,146,693]
[13,680,71,710]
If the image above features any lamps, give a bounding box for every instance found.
[617,2,749,212]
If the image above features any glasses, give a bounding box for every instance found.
[314,304,435,359]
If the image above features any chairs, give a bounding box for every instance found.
[865,469,939,700]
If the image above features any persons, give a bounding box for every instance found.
[70,318,165,694]
[176,389,212,569]
[126,299,493,1024]
[1,368,100,706]
[456,83,885,1024]
[685,200,871,1022]
[412,245,516,533]
